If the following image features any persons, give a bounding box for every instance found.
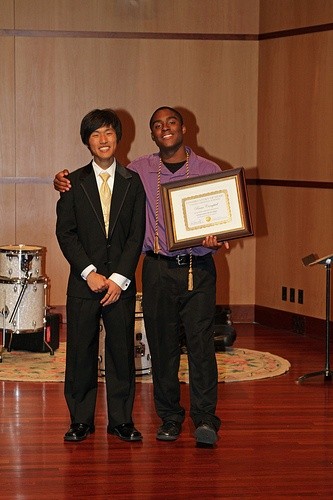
[54,106,229,445]
[56,108,147,442]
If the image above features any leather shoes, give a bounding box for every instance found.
[64,423,95,441]
[107,422,143,442]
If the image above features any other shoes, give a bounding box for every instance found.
[194,424,217,445]
[156,420,182,440]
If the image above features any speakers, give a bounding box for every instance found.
[3,313,61,353]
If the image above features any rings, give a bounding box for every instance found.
[107,292,111,295]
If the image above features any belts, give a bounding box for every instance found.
[147,252,211,266]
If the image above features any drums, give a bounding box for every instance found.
[95,292,152,377]
[0,276,48,334]
[0,245,46,280]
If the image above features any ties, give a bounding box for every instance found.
[99,173,112,239]
